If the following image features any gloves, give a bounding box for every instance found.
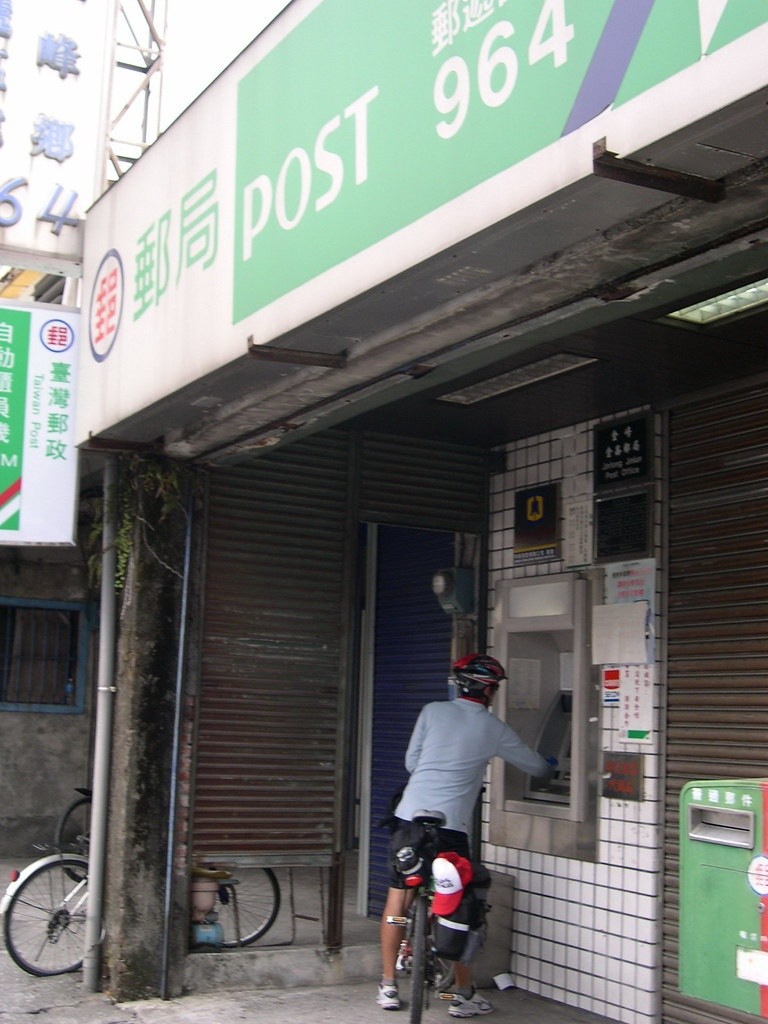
[546,756,559,766]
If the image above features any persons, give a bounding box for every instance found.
[377,653,559,1017]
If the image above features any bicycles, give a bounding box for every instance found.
[51,781,91,885]
[384,810,460,1024]
[0,842,281,977]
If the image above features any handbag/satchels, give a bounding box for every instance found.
[386,823,438,889]
[431,885,492,961]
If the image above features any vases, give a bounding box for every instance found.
[191,882,219,922]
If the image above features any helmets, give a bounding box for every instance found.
[452,653,508,692]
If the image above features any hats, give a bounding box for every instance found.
[431,851,474,915]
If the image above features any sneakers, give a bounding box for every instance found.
[448,985,494,1018]
[376,983,400,1010]
